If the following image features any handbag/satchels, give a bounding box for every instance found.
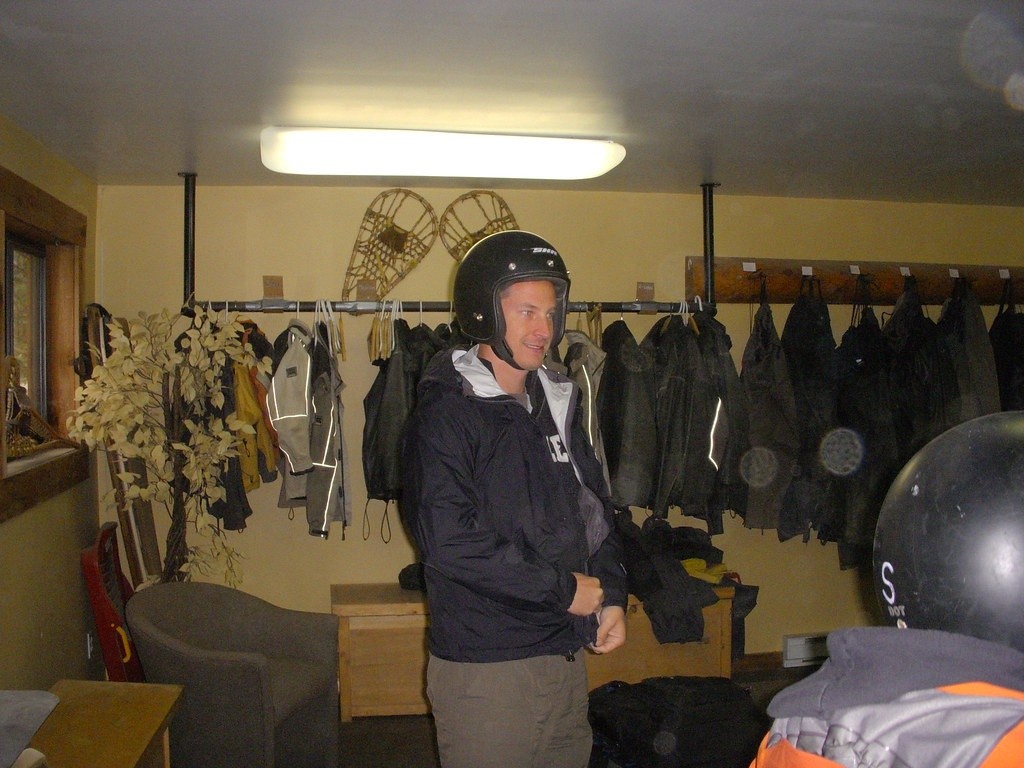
[587,676,770,768]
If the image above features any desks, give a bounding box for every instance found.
[6,681,186,768]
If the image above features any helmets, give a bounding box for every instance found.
[871,409,1024,653]
[454,230,572,350]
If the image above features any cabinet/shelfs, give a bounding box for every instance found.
[732,650,831,708]
[330,582,735,724]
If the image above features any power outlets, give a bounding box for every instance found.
[357,278,377,315]
[262,274,284,314]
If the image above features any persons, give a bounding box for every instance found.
[749,409,1024,768]
[396,231,630,768]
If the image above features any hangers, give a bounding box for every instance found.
[193,290,738,374]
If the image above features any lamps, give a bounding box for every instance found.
[258,125,629,180]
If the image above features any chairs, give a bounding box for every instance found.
[125,580,342,768]
